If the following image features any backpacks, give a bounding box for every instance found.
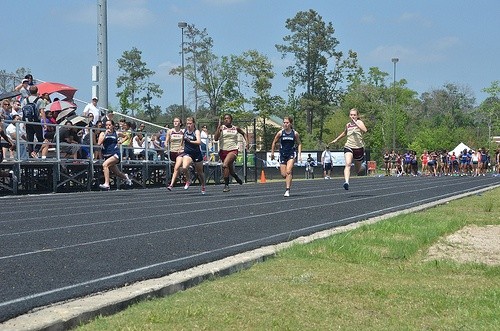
[22,97,40,122]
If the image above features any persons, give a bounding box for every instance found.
[98,120,131,189]
[321,146,333,179]
[271,116,302,196]
[22,86,47,158]
[201,125,208,160]
[40,91,169,162]
[383,146,500,178]
[164,117,194,192]
[330,108,368,190]
[307,154,315,179]
[0,74,38,162]
[177,117,206,196]
[214,114,250,192]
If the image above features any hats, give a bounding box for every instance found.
[92,97,98,100]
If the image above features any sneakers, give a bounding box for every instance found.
[284,189,290,197]
[223,186,229,192]
[167,185,173,191]
[384,170,500,178]
[99,184,110,189]
[184,181,191,189]
[343,182,349,190]
[201,186,206,195]
[31,151,38,158]
[125,174,131,185]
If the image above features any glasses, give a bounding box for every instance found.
[17,104,21,106]
[4,103,10,106]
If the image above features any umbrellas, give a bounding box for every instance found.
[56,107,75,122]
[43,100,78,112]
[0,92,22,101]
[59,115,89,133]
[30,82,79,100]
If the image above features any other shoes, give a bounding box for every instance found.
[324,176,331,179]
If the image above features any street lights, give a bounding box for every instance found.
[178,22,187,128]
[392,57,399,152]
